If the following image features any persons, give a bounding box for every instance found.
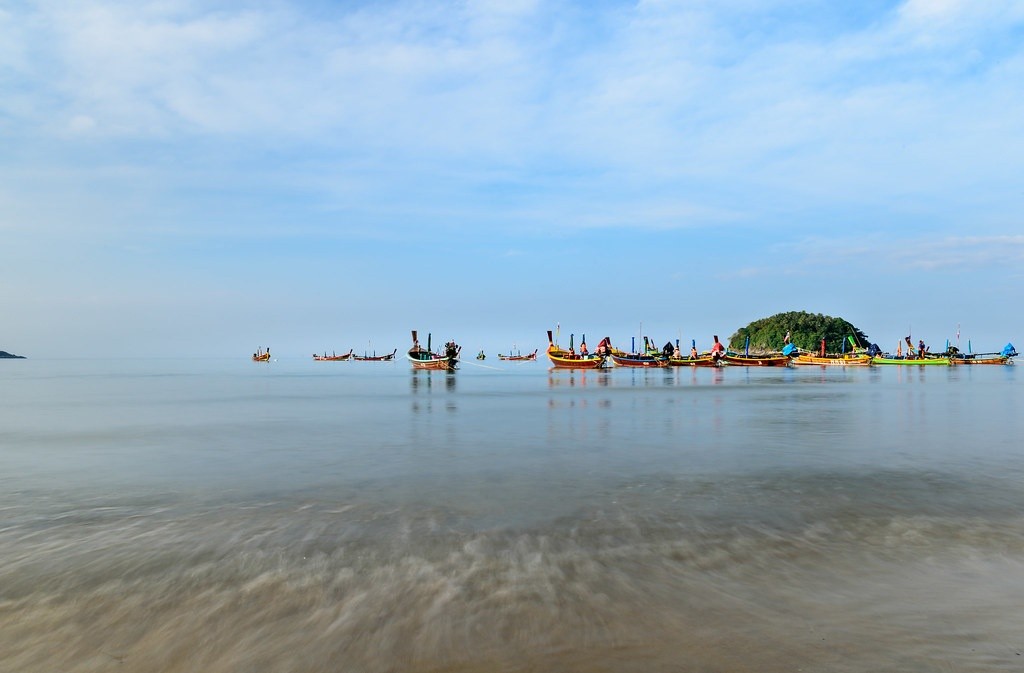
[687,347,697,360]
[918,340,925,360]
[563,348,574,359]
[674,346,683,360]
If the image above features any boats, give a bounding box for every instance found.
[670,353,725,366]
[313,349,353,361]
[956,346,1017,367]
[726,349,785,367]
[546,330,611,368]
[792,346,877,366]
[406,331,461,370]
[475,351,484,358]
[252,349,270,360]
[880,352,952,364]
[499,349,538,360]
[612,347,672,367]
[355,349,398,361]
